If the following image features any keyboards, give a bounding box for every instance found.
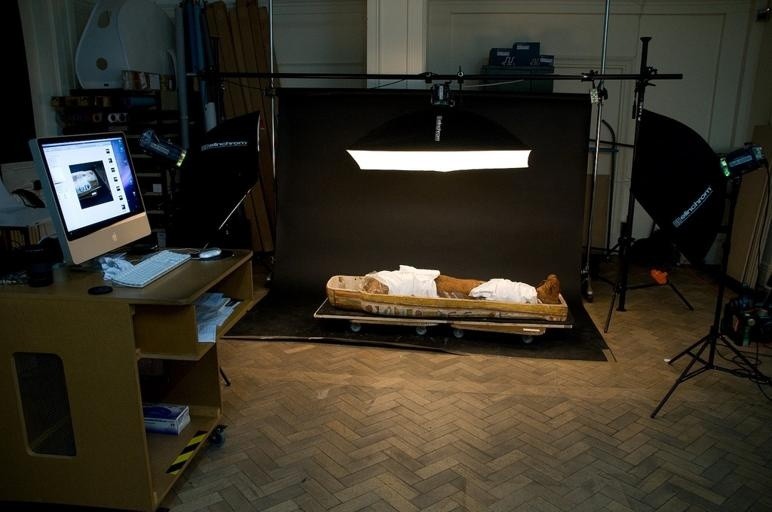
[107,249,192,290]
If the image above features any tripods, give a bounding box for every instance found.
[649,177,768,419]
[590,86,694,334]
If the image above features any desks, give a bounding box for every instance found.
[3,246,270,511]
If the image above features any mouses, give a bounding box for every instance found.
[199,247,223,261]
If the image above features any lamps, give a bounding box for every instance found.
[629,107,727,275]
[180,110,262,248]
[346,83,534,173]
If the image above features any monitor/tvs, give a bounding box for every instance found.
[28,131,153,274]
